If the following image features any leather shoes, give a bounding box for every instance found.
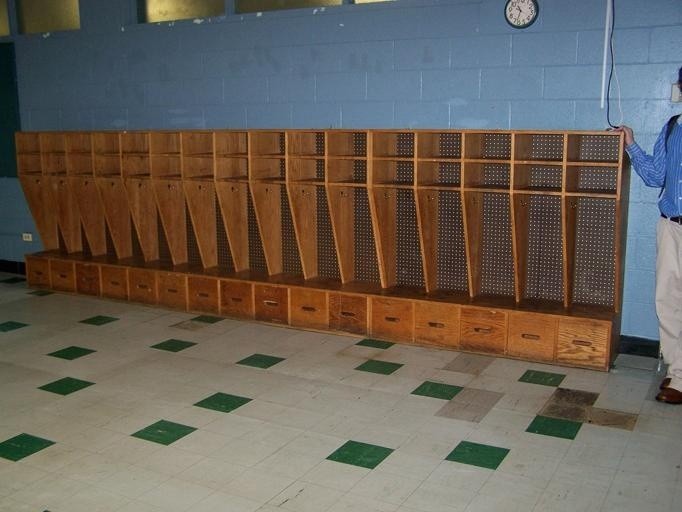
[655,378,682,404]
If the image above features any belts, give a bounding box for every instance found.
[661,211,682,225]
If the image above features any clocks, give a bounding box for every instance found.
[504,1,539,29]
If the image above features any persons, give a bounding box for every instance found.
[608,68,682,403]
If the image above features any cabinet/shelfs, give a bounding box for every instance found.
[14,129,630,374]
